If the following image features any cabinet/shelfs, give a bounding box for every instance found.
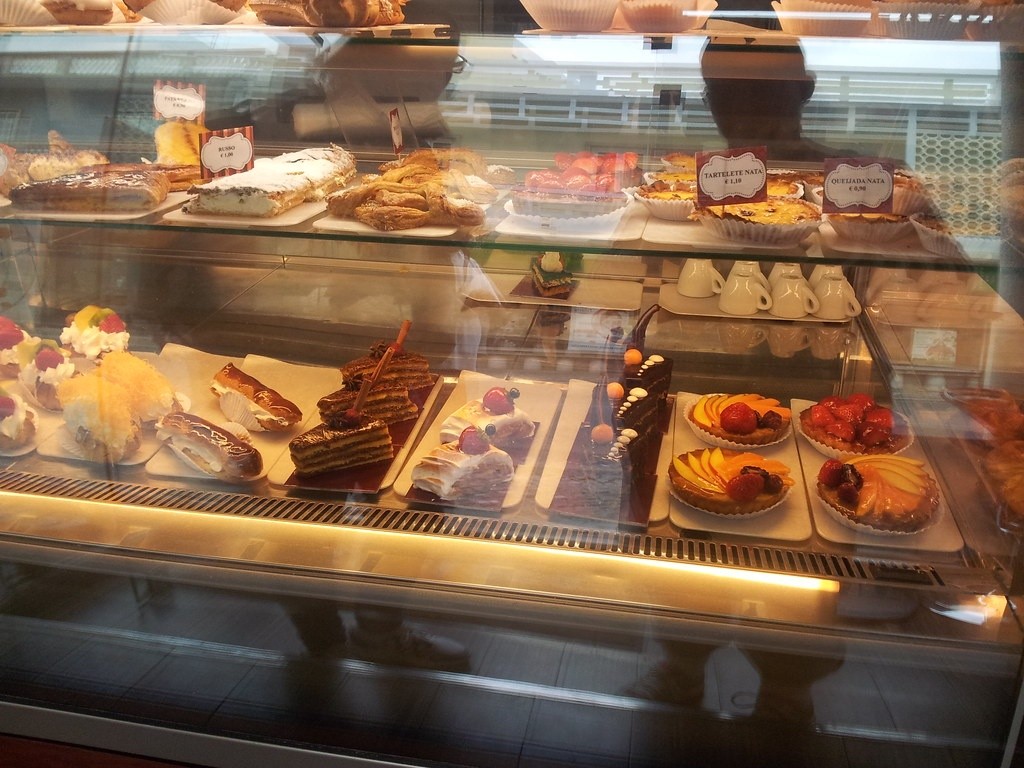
[0,23,1024,768]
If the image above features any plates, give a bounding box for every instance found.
[797,403,914,460]
[814,475,946,536]
[683,394,792,450]
[664,474,792,520]
[504,157,1014,260]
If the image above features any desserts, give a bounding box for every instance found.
[0,0,1024,534]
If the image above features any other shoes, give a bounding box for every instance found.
[389,629,472,669]
[326,647,373,664]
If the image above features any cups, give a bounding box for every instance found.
[680,320,857,360]
[677,258,862,319]
[866,267,995,325]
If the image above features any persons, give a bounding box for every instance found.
[610,33,975,734]
[125,24,471,718]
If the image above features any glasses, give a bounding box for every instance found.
[699,81,793,107]
[452,54,468,73]
